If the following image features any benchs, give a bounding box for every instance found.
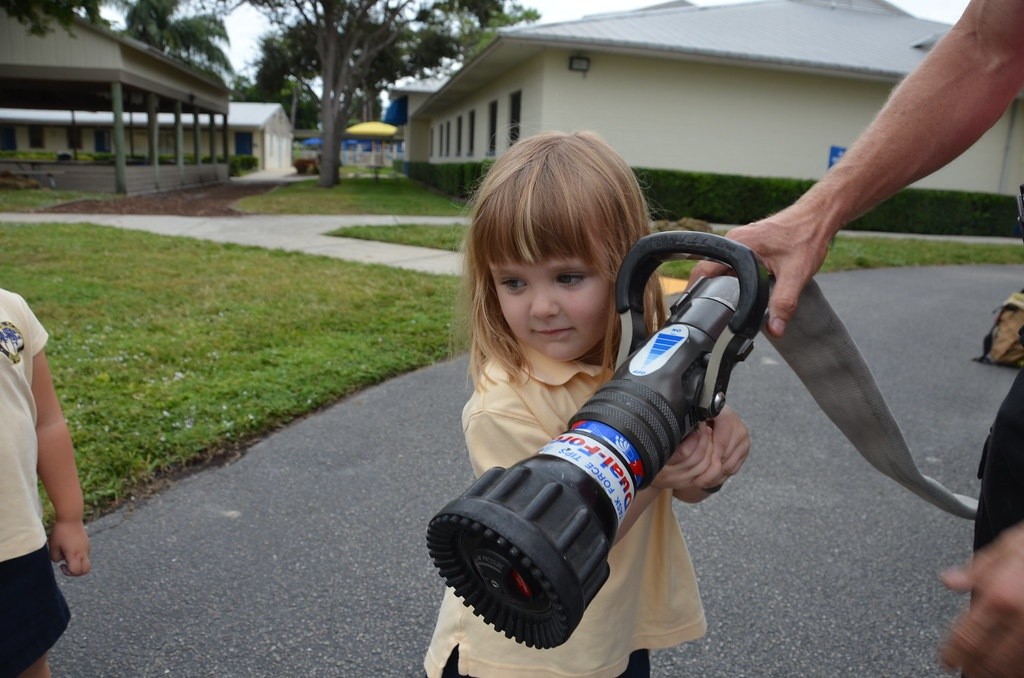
[8,168,69,189]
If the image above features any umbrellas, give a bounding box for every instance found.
[346,121,397,164]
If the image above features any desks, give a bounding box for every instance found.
[0,156,57,168]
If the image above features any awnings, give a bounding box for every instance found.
[385,96,408,124]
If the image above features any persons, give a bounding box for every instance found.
[685,1,1024,677]
[418,133,747,675]
[0,276,92,678]
